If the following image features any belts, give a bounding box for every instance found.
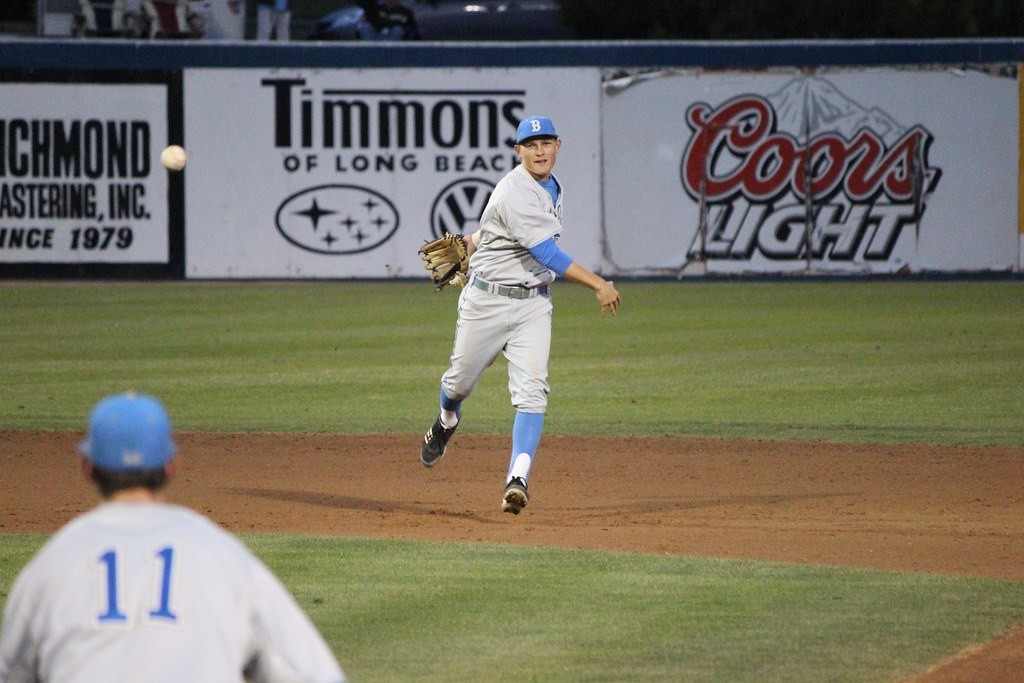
[472,275,547,299]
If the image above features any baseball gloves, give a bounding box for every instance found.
[420,232,468,292]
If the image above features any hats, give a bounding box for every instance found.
[516,116,558,145]
[78,389,180,476]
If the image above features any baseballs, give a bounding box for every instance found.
[162,145,187,170]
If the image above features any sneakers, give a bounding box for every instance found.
[420,409,462,469]
[501,476,529,515]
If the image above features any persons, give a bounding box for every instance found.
[1,387,350,682]
[72,0,296,44]
[417,116,622,514]
